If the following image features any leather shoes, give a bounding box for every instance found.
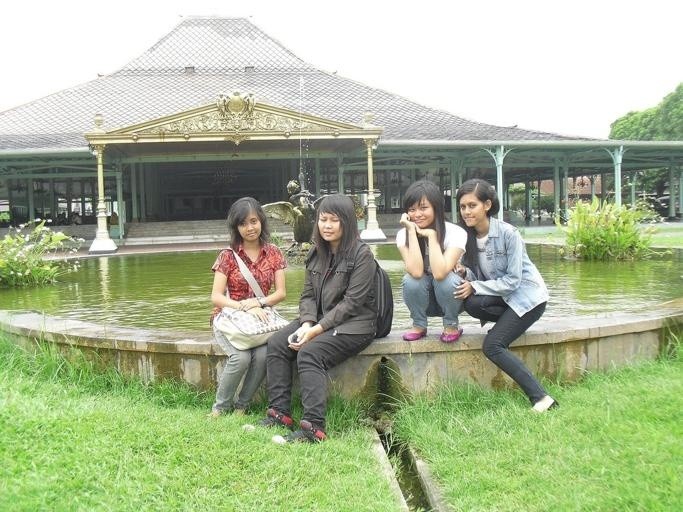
[440,327,463,344]
[402,329,430,341]
[528,399,561,415]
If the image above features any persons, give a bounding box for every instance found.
[209,197,288,418]
[0,207,82,227]
[242,193,378,447]
[395,179,469,343]
[454,178,560,416]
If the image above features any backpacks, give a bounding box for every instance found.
[346,242,395,339]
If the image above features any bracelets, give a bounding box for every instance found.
[257,296,267,308]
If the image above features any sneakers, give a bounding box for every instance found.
[231,409,246,418]
[206,408,221,417]
[271,417,329,447]
[242,409,294,436]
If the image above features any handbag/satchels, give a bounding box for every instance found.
[213,303,291,351]
